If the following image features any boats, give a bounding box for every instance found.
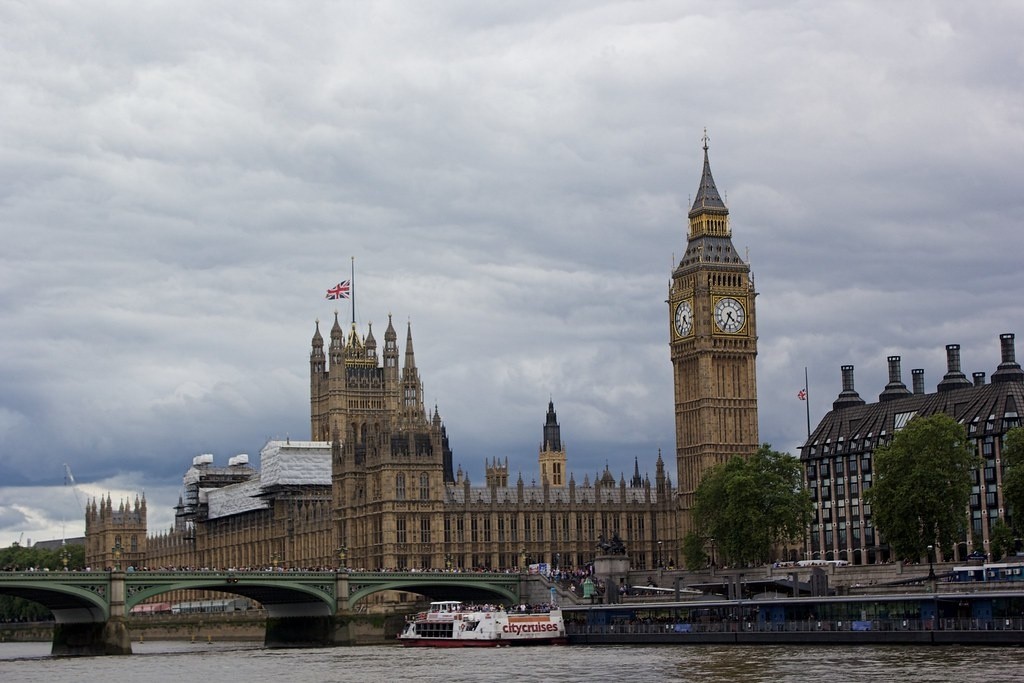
[395,601,569,648]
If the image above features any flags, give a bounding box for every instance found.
[798,388,808,400]
[326,280,350,299]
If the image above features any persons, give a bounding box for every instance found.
[0,557,1024,632]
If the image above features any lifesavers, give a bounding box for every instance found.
[461,624,464,629]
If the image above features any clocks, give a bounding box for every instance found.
[673,300,693,338]
[714,297,746,334]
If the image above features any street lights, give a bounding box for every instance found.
[444,553,453,573]
[335,545,348,572]
[710,539,716,566]
[927,546,935,576]
[112,543,125,571]
[60,548,72,572]
[657,541,664,567]
